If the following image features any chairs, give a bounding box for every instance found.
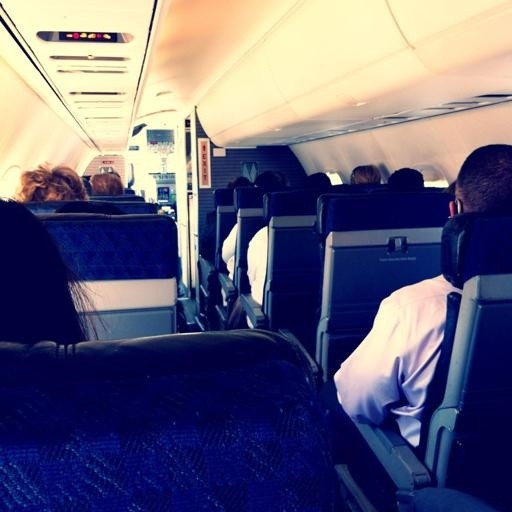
[279,190,448,384]
[36,211,178,341]
[336,273,512,510]
[195,187,233,331]
[24,201,157,214]
[88,195,145,201]
[215,187,268,329]
[241,192,320,328]
[0,327,335,511]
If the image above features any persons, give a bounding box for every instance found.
[0,198,110,342]
[199,164,424,329]
[89,170,123,195]
[333,143,512,450]
[13,164,89,204]
